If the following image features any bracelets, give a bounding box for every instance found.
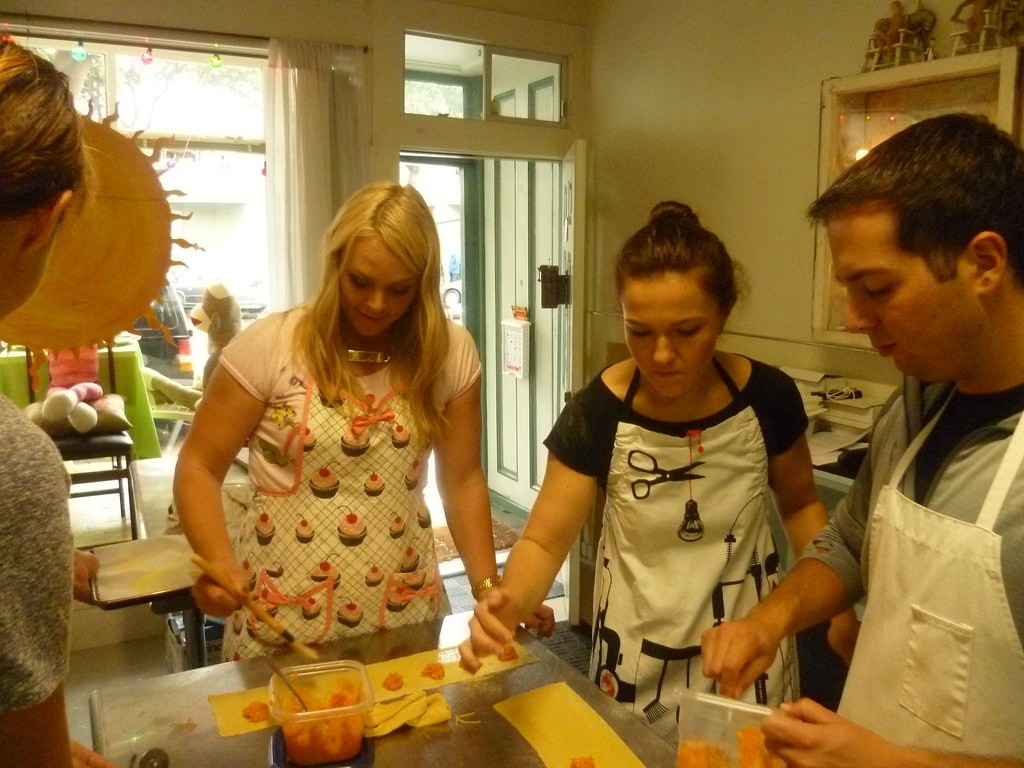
[471,575,501,598]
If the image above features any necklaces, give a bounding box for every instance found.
[340,333,398,363]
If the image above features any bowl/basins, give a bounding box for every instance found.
[266,660,376,766]
[677,688,789,767]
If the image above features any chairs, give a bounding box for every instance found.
[861,28,919,74]
[27,337,138,542]
[949,1,1015,57]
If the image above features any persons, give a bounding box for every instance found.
[457,202,860,751]
[173,182,554,664]
[0,61,112,768]
[701,111,1024,768]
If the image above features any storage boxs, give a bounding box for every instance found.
[268,661,376,764]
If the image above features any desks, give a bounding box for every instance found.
[88,609,682,768]
[0,330,163,461]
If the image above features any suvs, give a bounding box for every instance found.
[130,280,194,387]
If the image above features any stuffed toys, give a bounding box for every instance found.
[145,285,241,413]
[41,340,103,434]
